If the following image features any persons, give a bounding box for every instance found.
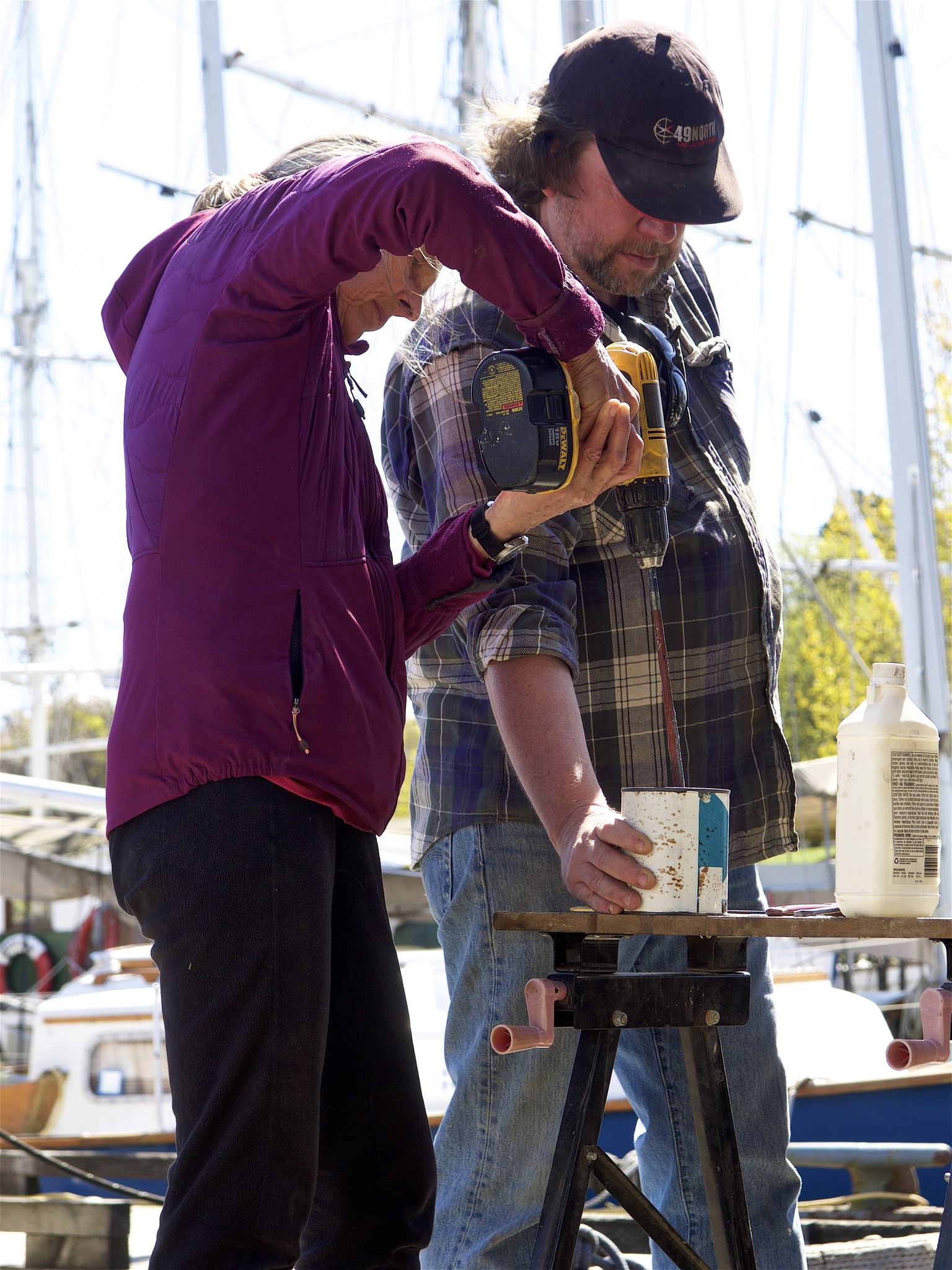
[98,132,647,1270]
[384,28,812,1270]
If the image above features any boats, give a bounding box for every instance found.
[10,927,952,1243]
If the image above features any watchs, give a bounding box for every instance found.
[471,500,528,564]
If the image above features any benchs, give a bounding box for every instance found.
[489,905,951,1270]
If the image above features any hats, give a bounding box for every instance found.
[550,22,743,224]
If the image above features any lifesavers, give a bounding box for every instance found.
[67,903,118,979]
[0,932,55,999]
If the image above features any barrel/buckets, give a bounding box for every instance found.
[617,784,736,915]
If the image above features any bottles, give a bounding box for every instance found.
[834,660,940,918]
